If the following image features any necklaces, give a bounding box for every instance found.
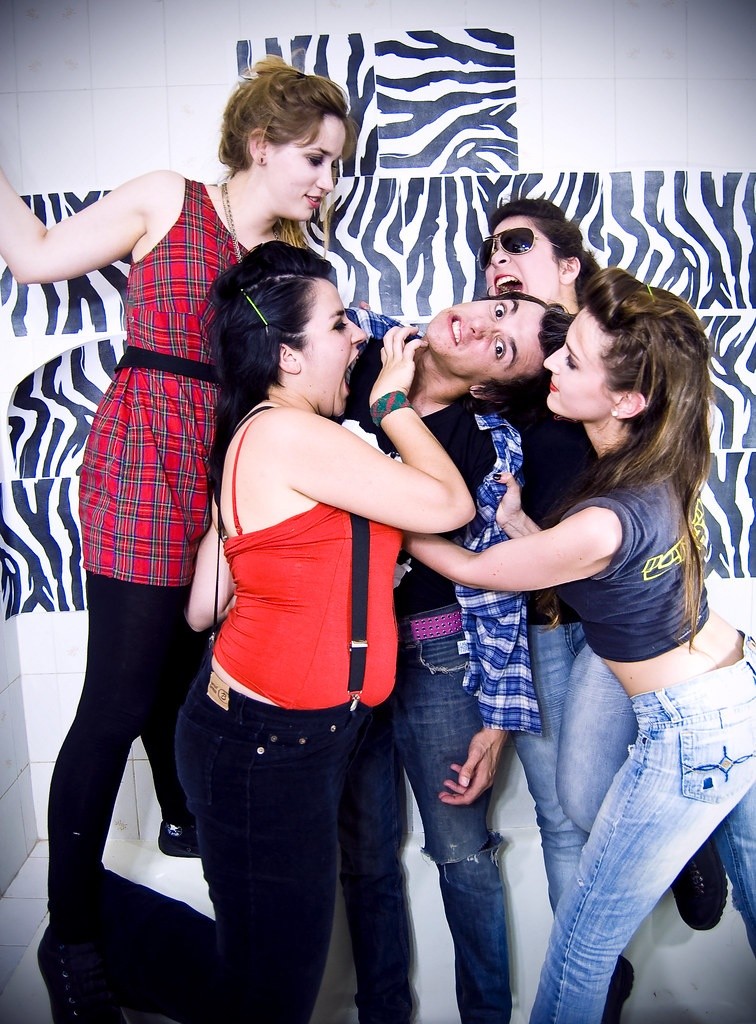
[221,182,281,266]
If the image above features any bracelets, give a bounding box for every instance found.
[369,391,414,427]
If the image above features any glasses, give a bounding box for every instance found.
[476,227,561,271]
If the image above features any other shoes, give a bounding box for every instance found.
[158,821,202,857]
[671,837,729,931]
[38,926,127,1024]
[601,955,634,1024]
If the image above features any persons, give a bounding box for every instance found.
[0,54,349,1024]
[184,198,756,1024]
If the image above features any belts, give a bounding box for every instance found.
[397,609,463,643]
[117,346,222,385]
